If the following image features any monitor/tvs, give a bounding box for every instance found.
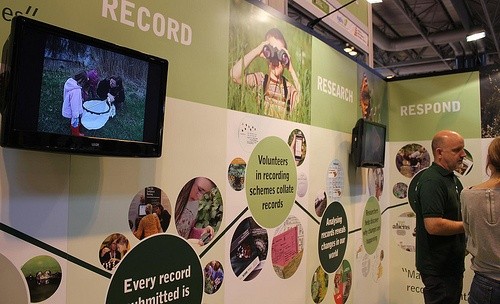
[138,204,146,217]
[0,16,169,156]
[351,118,387,168]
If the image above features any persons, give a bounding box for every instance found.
[129,202,171,239]
[334,272,351,304]
[203,261,224,294]
[231,240,257,277]
[231,28,303,120]
[396,147,430,176]
[290,134,304,165]
[415,130,467,304]
[100,233,130,270]
[62,68,125,137]
[460,136,500,304]
[175,177,215,240]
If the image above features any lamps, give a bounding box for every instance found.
[466,26,487,42]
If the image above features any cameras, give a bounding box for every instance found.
[199,233,211,245]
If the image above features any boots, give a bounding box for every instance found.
[70,124,85,136]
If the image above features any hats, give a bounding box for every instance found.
[87,70,98,79]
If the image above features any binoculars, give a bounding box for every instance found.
[262,44,289,67]
